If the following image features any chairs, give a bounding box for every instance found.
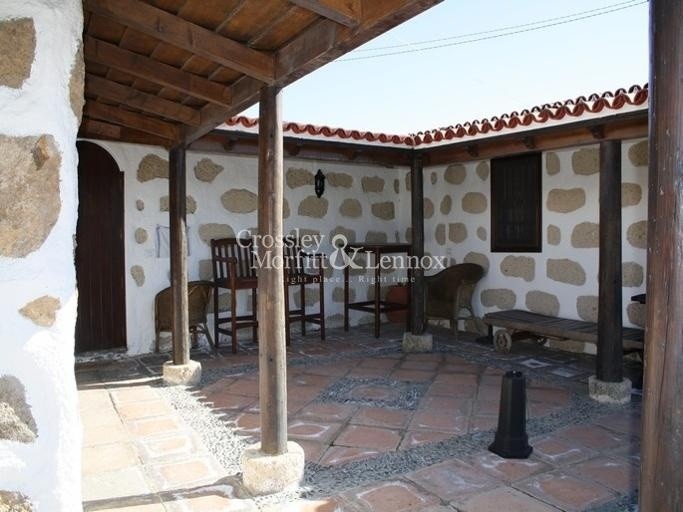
[155,281,216,354]
[283,236,326,346]
[423,264,484,339]
[209,237,259,354]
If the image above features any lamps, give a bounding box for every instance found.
[315,169,325,198]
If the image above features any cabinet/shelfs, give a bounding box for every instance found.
[341,242,413,337]
